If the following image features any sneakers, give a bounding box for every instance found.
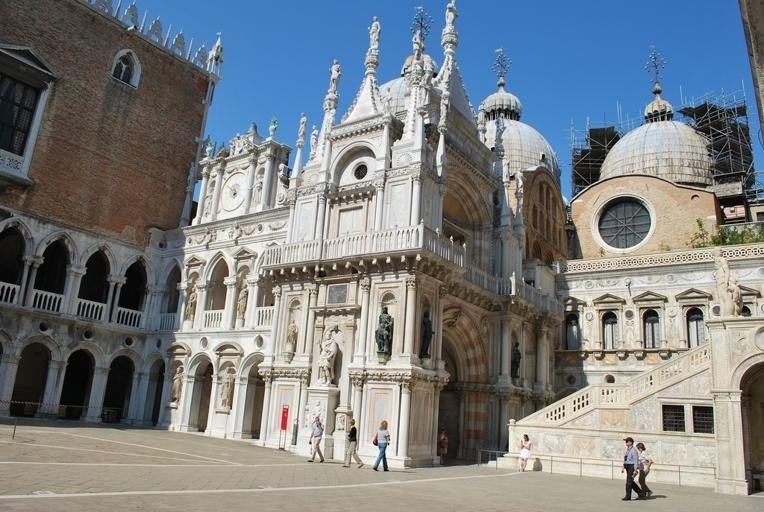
[623,492,652,500]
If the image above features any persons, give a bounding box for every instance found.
[518,434,531,472]
[375,307,392,354]
[219,367,235,408]
[236,284,249,319]
[288,318,298,344]
[713,247,743,316]
[420,311,432,358]
[512,342,521,378]
[373,421,390,471]
[637,442,652,498]
[620,436,645,500]
[308,417,324,462]
[343,419,363,468]
[437,428,448,465]
[318,323,343,386]
[185,288,195,321]
[169,367,184,402]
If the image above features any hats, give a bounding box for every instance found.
[623,437,633,442]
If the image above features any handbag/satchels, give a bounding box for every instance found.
[372,437,378,446]
